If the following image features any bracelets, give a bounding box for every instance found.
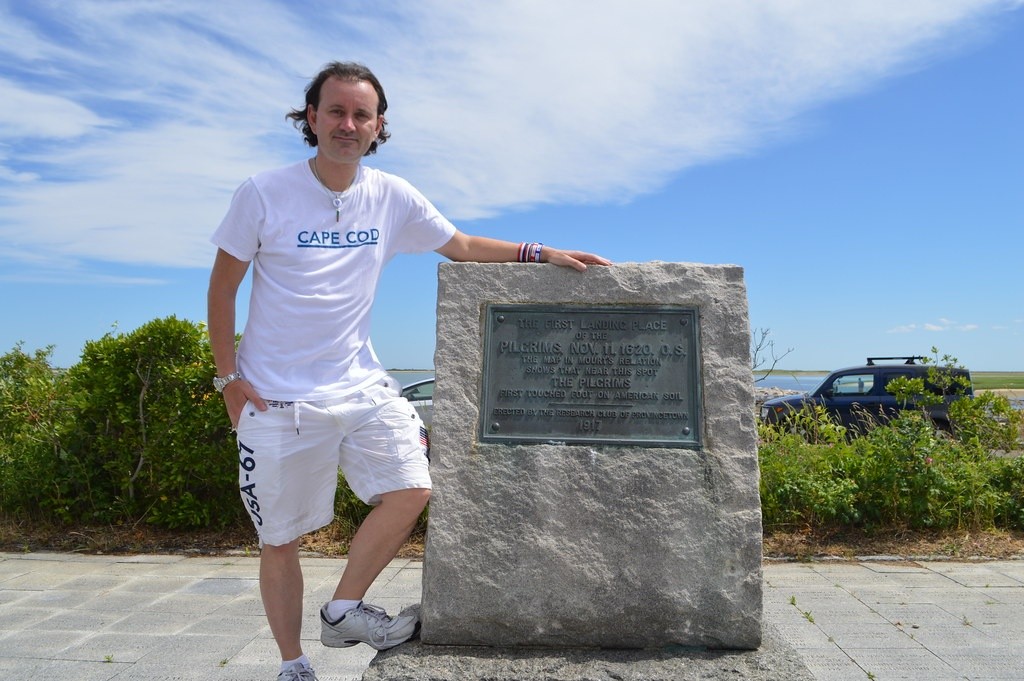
[518,242,544,263]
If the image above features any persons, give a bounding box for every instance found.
[206,63,613,681]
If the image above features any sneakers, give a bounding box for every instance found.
[277,663,319,681]
[320,601,419,651]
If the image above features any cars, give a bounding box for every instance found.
[400,377,434,429]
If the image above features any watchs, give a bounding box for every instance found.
[213,371,241,394]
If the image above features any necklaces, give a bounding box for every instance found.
[311,156,365,221]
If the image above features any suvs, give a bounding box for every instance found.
[757,354,976,444]
